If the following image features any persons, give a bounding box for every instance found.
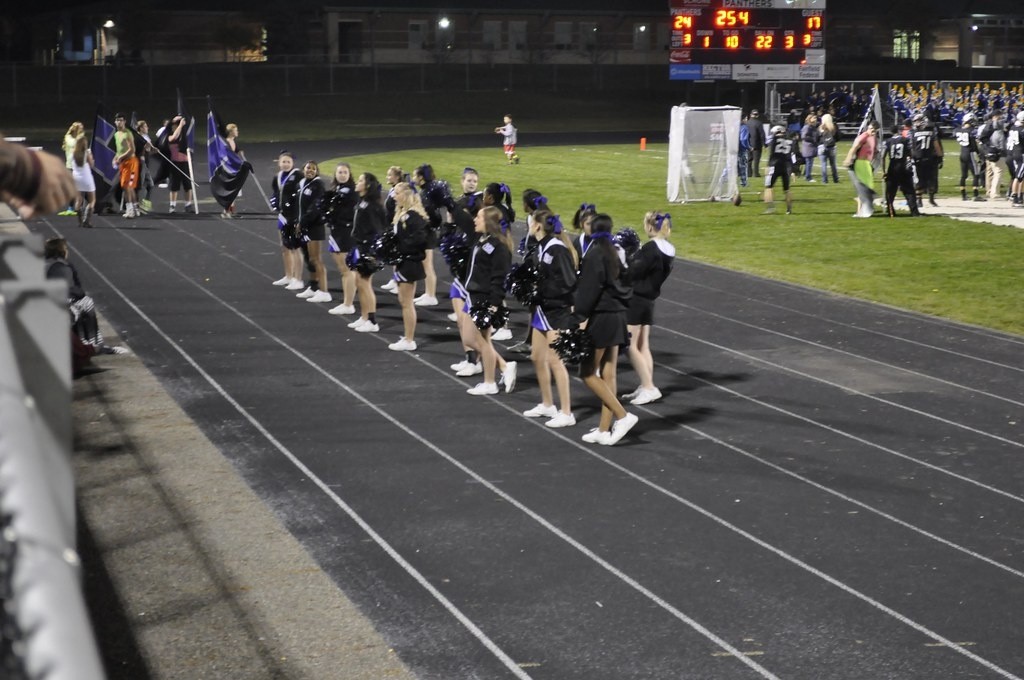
[270,152,676,445]
[720,83,1024,216]
[220,123,248,219]
[498,115,519,165]
[46,237,117,354]
[56,118,196,228]
[0,134,77,221]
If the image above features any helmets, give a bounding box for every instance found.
[1017,111,1024,123]
[962,113,979,125]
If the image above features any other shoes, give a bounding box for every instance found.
[737,167,1024,220]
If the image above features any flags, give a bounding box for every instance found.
[207,98,256,212]
[86,110,196,214]
[856,88,887,173]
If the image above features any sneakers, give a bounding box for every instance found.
[609,412,639,445]
[389,335,417,351]
[415,296,439,306]
[271,270,379,333]
[582,427,611,445]
[498,361,517,393]
[466,381,499,396]
[629,386,662,405]
[621,384,643,398]
[448,313,459,322]
[507,341,533,353]
[54,192,241,227]
[455,361,483,376]
[490,328,513,341]
[413,293,428,302]
[450,359,468,371]
[523,402,557,417]
[381,279,398,289]
[545,410,576,428]
[389,286,399,295]
